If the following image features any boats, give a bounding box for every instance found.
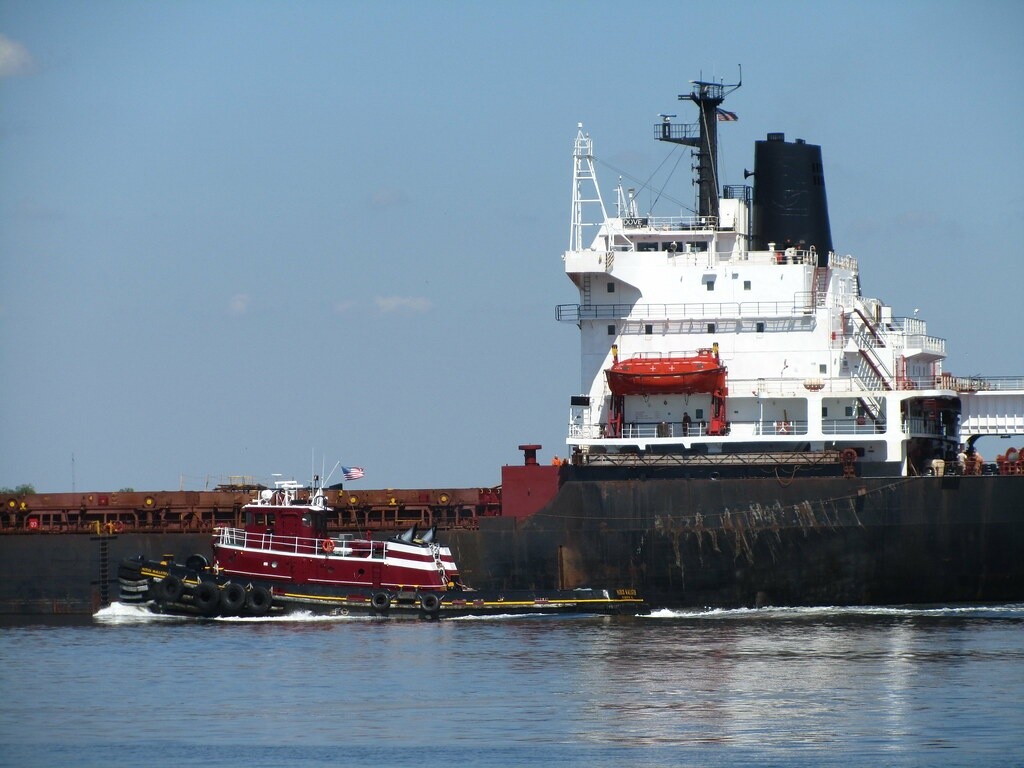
[1,63,1024,611]
[94,457,650,627]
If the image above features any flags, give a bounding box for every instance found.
[341,465,364,481]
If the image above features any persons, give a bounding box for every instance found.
[682,412,692,436]
[944,447,984,475]
[552,454,567,465]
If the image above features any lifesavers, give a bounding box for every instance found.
[371,592,391,611]
[159,575,184,602]
[420,593,439,612]
[220,582,246,611]
[246,586,272,613]
[322,539,335,552]
[192,581,220,609]
